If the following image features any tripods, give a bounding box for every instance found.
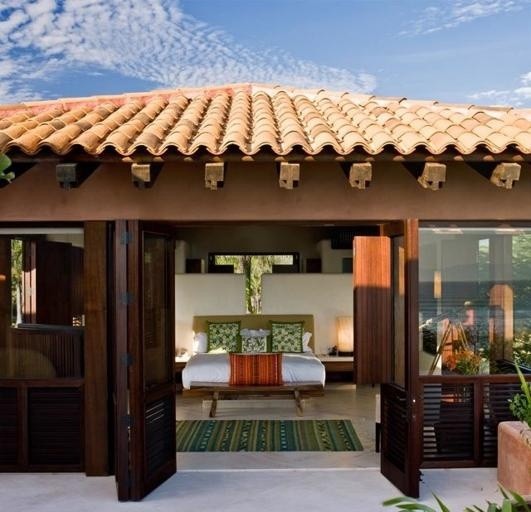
[427,314,496,421]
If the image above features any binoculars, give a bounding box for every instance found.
[419,306,464,328]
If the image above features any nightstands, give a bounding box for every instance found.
[316,353,356,385]
[175,354,195,393]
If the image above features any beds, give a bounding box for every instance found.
[182,314,328,419]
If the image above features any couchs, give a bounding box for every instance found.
[497,421,530,501]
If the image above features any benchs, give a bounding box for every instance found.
[192,379,327,417]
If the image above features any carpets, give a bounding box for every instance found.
[176,417,366,453]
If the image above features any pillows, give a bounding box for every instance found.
[269,319,306,353]
[237,328,271,338]
[207,320,241,353]
[239,335,272,352]
[229,352,284,386]
[303,331,311,352]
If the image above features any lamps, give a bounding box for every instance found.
[336,315,355,357]
